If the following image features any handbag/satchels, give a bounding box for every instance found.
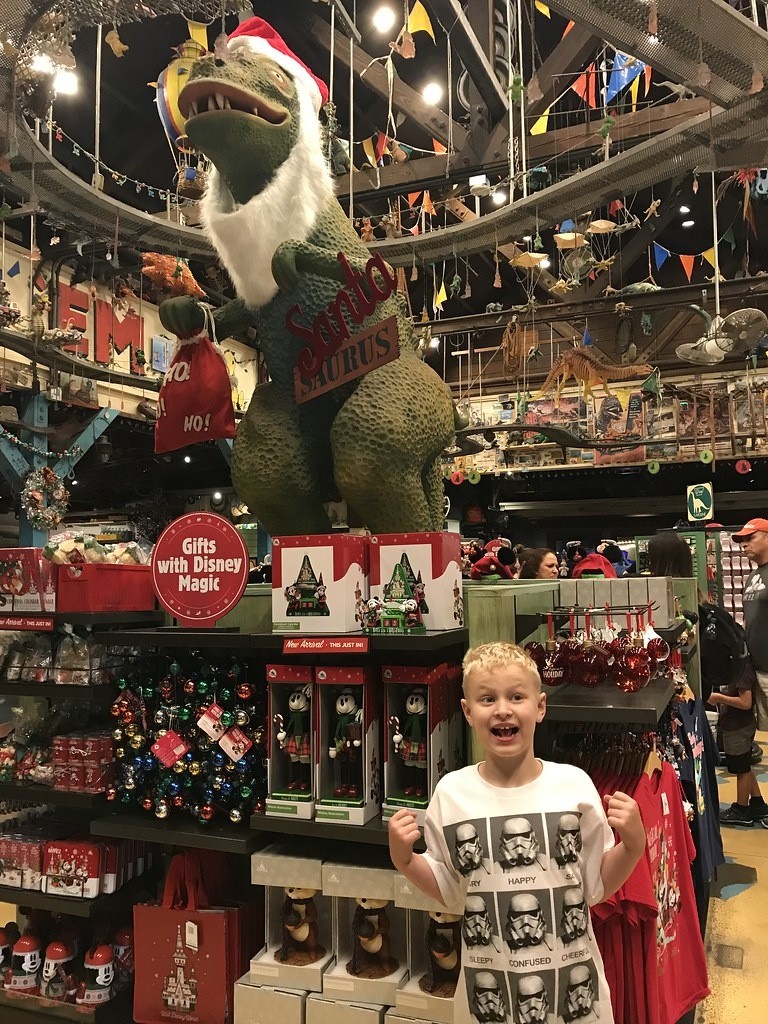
[133,846,258,1024]
[153,298,242,455]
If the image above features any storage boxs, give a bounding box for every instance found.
[54,726,117,793]
[0,546,155,612]
[233,844,462,1024]
[1,803,156,899]
[265,664,465,824]
[559,576,675,630]
[270,531,464,632]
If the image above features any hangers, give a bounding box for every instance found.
[552,720,664,778]
[683,680,696,705]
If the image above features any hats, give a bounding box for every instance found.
[470,540,519,581]
[197,15,328,310]
[732,518,768,543]
[573,553,617,578]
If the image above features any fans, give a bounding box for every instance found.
[715,307,768,353]
[676,335,724,366]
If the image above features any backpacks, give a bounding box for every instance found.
[698,602,749,684]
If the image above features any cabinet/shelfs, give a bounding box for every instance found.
[616,524,759,630]
[0,576,704,1024]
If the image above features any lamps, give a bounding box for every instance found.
[706,172,734,355]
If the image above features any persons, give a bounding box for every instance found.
[389,642,645,1024]
[707,657,768,829]
[731,518,768,731]
[461,544,536,579]
[519,548,559,579]
[648,532,712,703]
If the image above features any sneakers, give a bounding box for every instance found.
[718,803,753,826]
[747,803,768,823]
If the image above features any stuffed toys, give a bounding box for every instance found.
[275,887,320,964]
[425,910,461,993]
[351,898,390,975]
